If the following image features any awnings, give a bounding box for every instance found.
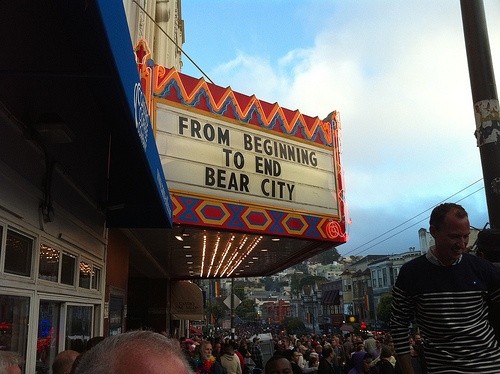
[0,1,171,228]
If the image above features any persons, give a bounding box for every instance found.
[180,325,421,374]
[72,331,194,374]
[389,202,500,374]
[52,350,80,374]
[265,355,293,374]
[0,350,21,374]
